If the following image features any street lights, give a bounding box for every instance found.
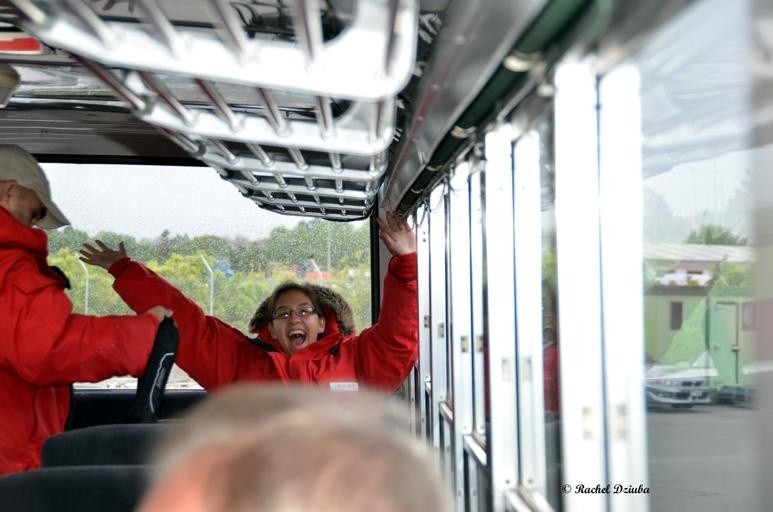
[199,254,214,319]
[309,258,324,285]
[75,253,89,315]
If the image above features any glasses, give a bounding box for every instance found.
[272,305,322,321]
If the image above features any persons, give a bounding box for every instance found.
[77,210,418,402]
[0,142,179,476]
[131,381,453,512]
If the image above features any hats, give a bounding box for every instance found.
[0,142,72,231]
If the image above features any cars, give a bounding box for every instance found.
[645,368,711,407]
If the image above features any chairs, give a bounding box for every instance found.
[0,422,184,510]
